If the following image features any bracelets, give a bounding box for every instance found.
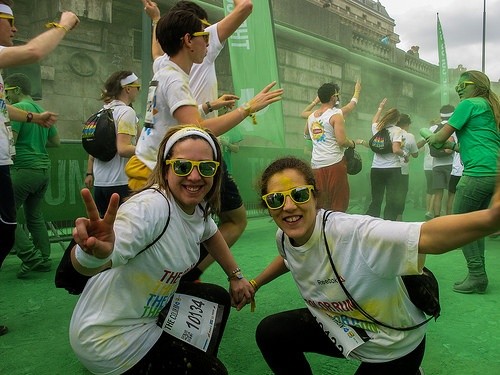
[351,98,358,104]
[250,280,259,291]
[312,101,316,106]
[47,21,68,33]
[378,108,382,110]
[206,101,213,112]
[75,243,114,268]
[244,104,257,125]
[452,143,456,150]
[86,173,93,176]
[228,267,241,279]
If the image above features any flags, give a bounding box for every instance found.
[223,1,285,148]
[437,19,449,105]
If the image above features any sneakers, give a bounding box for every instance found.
[425,212,434,219]
[16,254,44,276]
[32,259,52,272]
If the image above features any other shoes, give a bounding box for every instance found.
[453,272,488,294]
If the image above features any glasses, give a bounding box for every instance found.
[4,87,17,95]
[262,185,314,209]
[200,19,211,28]
[166,159,220,177]
[124,84,141,91]
[455,81,476,91]
[0,14,14,27]
[181,32,209,44]
[334,93,339,98]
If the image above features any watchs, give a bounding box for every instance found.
[26,112,33,123]
[228,272,243,282]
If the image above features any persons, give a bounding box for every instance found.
[299,70,500,292]
[69,124,254,375]
[125,0,285,282]
[381,34,419,58]
[86,71,142,218]
[232,157,500,375]
[0,0,81,336]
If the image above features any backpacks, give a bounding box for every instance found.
[430,124,452,157]
[282,209,440,331]
[55,188,205,295]
[369,126,393,154]
[82,104,129,162]
